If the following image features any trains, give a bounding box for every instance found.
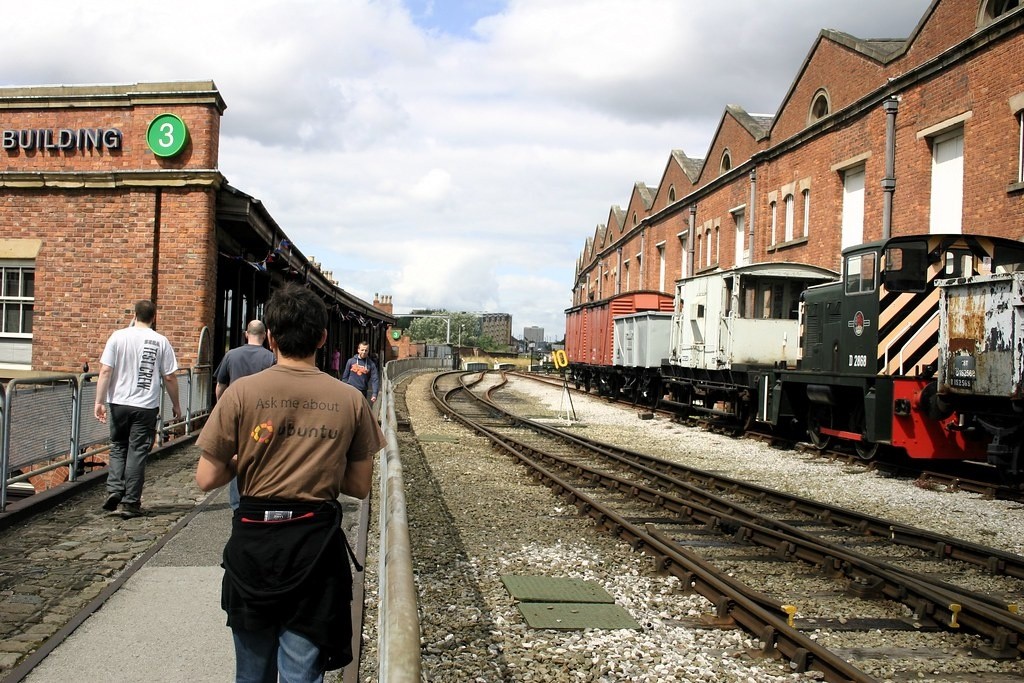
[564,232,1024,475]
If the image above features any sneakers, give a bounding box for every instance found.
[103,492,122,511]
[121,503,158,517]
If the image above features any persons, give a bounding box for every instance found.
[195,284,387,683]
[213,319,280,512]
[330,348,340,378]
[341,342,379,403]
[94,301,182,518]
